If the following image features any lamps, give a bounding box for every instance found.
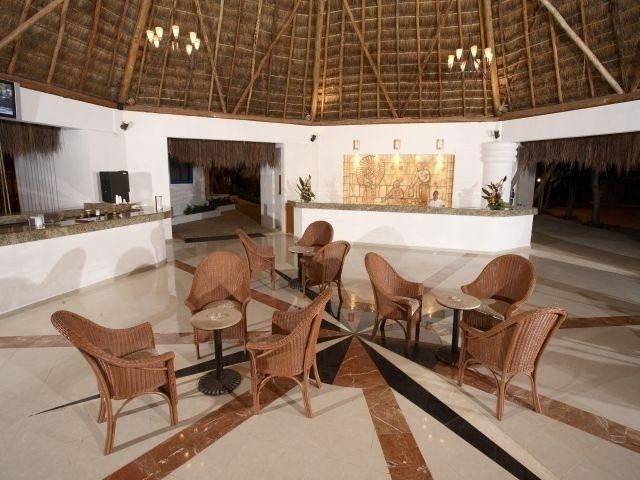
[447,0,494,80]
[494,130,500,138]
[121,121,129,130]
[146,0,201,56]
[310,134,316,141]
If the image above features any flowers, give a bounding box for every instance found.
[481,176,511,210]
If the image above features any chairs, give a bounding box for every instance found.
[245,285,334,418]
[185,250,253,361]
[364,252,425,356]
[295,221,334,277]
[303,240,351,303]
[458,307,568,422]
[235,228,277,290]
[461,254,537,354]
[51,310,178,455]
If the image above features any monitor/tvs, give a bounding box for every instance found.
[0,78,21,121]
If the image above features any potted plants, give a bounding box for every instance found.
[295,174,316,204]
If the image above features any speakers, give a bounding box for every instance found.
[100,171,129,203]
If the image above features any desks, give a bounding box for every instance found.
[436,290,481,368]
[288,245,319,290]
[190,307,242,395]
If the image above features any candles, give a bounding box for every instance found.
[35,217,44,230]
[96,209,100,216]
